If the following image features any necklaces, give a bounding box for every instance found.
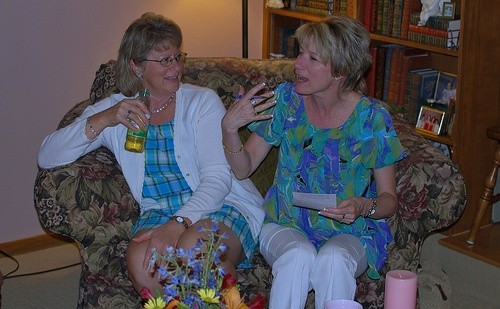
[150,94,174,115]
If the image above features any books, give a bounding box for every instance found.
[281,0,460,123]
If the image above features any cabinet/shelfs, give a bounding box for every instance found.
[261,0,500,237]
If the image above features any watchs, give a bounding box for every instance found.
[363,198,377,219]
[170,216,189,229]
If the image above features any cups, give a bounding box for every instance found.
[384,270,417,309]
[325,299,363,309]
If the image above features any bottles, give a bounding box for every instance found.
[124,88,150,153]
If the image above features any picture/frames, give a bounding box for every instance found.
[415,106,446,136]
[433,70,457,105]
[442,2,456,19]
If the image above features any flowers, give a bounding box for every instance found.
[140,214,266,309]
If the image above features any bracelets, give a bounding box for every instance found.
[222,140,243,154]
[87,116,99,137]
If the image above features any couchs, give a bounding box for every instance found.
[34,56,468,309]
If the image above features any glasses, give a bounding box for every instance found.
[139,51,188,68]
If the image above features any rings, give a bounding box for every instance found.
[126,109,132,118]
[340,214,345,223]
[251,99,258,116]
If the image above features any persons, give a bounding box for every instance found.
[445,7,452,14]
[418,115,439,132]
[440,80,456,104]
[222,17,407,309]
[37,10,264,298]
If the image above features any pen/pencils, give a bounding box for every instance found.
[234,94,267,99]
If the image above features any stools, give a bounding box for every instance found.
[466,126,500,245]
